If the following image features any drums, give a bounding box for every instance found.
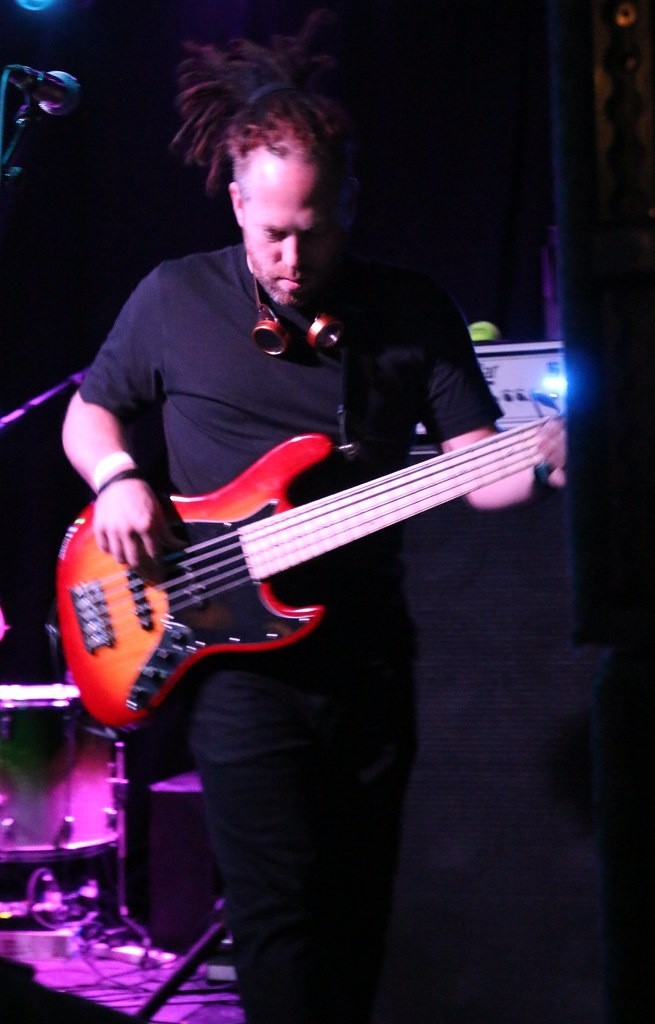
[0,857,102,921]
[0,683,121,865]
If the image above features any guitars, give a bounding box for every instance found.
[51,411,565,739]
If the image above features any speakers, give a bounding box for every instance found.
[381,439,622,1024]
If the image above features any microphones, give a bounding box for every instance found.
[2,65,81,116]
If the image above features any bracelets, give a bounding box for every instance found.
[98,469,146,494]
[92,449,133,491]
[531,463,553,484]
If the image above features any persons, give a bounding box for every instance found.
[60,6,570,1024]
